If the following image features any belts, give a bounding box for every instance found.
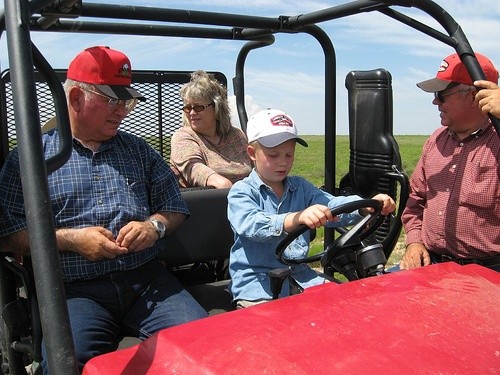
[426,250,500,266]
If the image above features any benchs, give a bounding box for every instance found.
[119,183,316,337]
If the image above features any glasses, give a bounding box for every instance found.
[77,86,134,108]
[182,102,214,113]
[434,87,472,103]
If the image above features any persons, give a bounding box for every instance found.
[228,108,396,310]
[170,69,256,189]
[0,46,209,375]
[401,51,500,273]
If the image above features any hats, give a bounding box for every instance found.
[66,46,147,102]
[416,52,500,92]
[246,108,308,148]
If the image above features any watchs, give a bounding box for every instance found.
[145,218,165,238]
